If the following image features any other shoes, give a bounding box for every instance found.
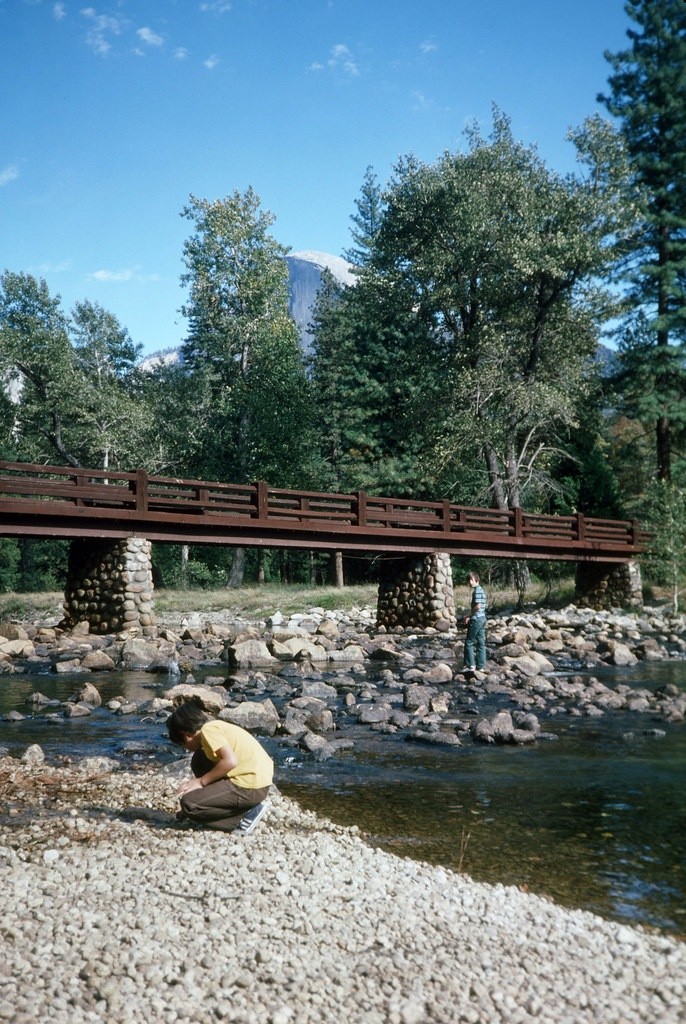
[479,668,485,672]
[461,665,476,672]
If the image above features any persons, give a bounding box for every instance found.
[461,572,489,673]
[166,700,274,837]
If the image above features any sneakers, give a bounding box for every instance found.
[237,803,266,833]
